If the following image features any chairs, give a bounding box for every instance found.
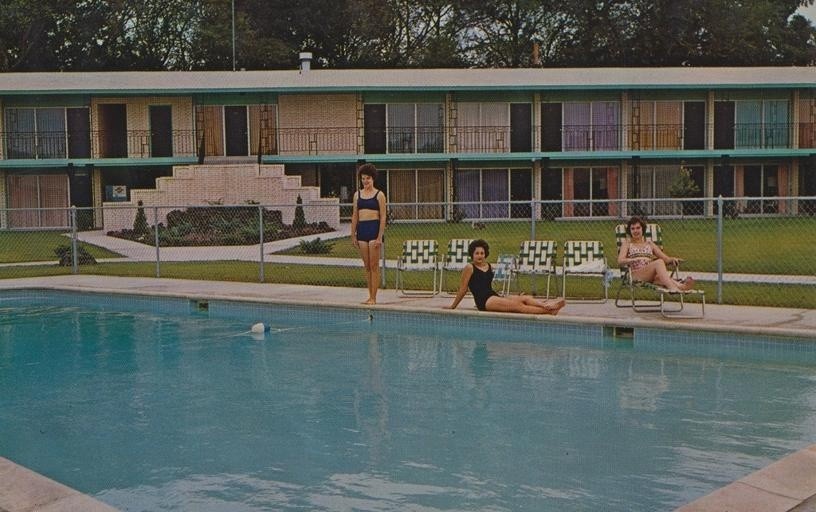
[440,239,486,298]
[561,241,608,303]
[395,240,440,298]
[505,240,557,302]
[615,224,705,319]
[489,253,515,296]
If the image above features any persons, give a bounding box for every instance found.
[450,339,570,412]
[440,239,566,316]
[351,163,386,305]
[352,331,389,474]
[617,216,693,293]
